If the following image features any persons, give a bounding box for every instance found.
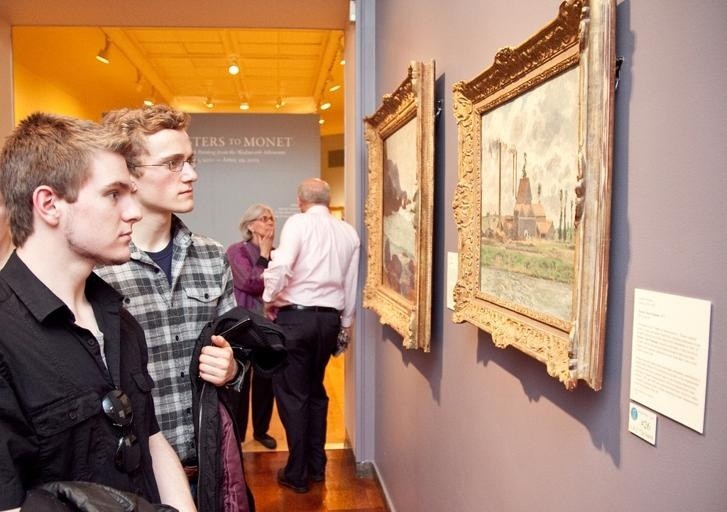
[0,113,197,511]
[262,178,362,493]
[225,203,279,451]
[91,103,248,511]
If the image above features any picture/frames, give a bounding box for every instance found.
[449,0,618,397]
[327,205,345,220]
[360,56,436,355]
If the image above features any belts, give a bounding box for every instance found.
[277,305,339,314]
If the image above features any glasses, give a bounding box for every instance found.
[102,390,142,472]
[134,157,197,171]
[250,216,274,221]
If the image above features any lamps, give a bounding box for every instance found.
[95,35,157,107]
[315,36,350,125]
[228,61,251,110]
[203,95,213,109]
[273,96,282,109]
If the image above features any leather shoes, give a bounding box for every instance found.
[277,469,309,492]
[257,433,277,448]
